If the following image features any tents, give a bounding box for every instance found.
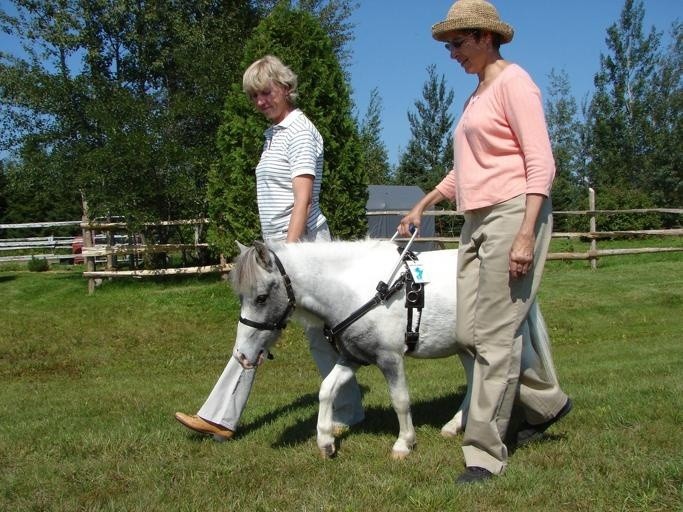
[362,185,436,252]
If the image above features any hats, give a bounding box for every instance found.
[430,1,514,45]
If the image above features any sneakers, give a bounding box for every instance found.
[518,396,572,441]
[174,410,234,442]
[332,424,356,441]
[456,465,498,486]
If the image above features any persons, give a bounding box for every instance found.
[173,54,366,444]
[397,0,574,486]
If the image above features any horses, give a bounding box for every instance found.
[230,238,559,462]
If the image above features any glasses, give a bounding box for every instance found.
[439,25,480,50]
